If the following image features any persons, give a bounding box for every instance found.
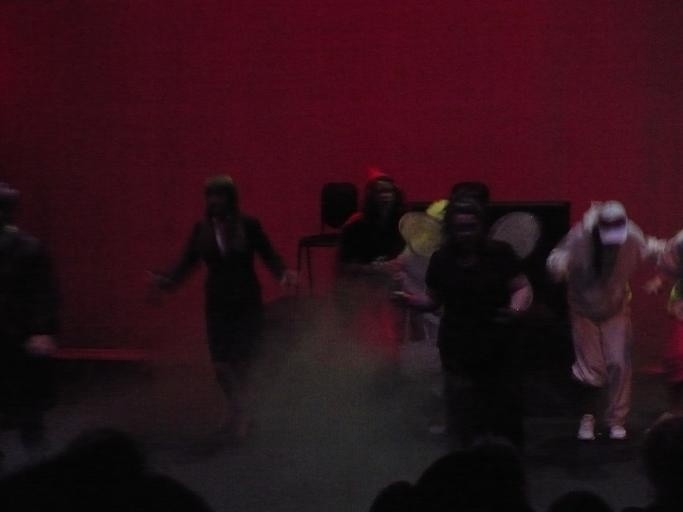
[337,177,683,512]
[1,191,64,452]
[160,173,291,399]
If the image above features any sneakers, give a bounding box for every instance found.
[577,414,598,441]
[610,424,627,440]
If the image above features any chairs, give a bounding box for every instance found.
[296,182,357,301]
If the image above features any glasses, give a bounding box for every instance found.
[450,204,481,214]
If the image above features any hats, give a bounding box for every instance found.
[598,201,628,246]
